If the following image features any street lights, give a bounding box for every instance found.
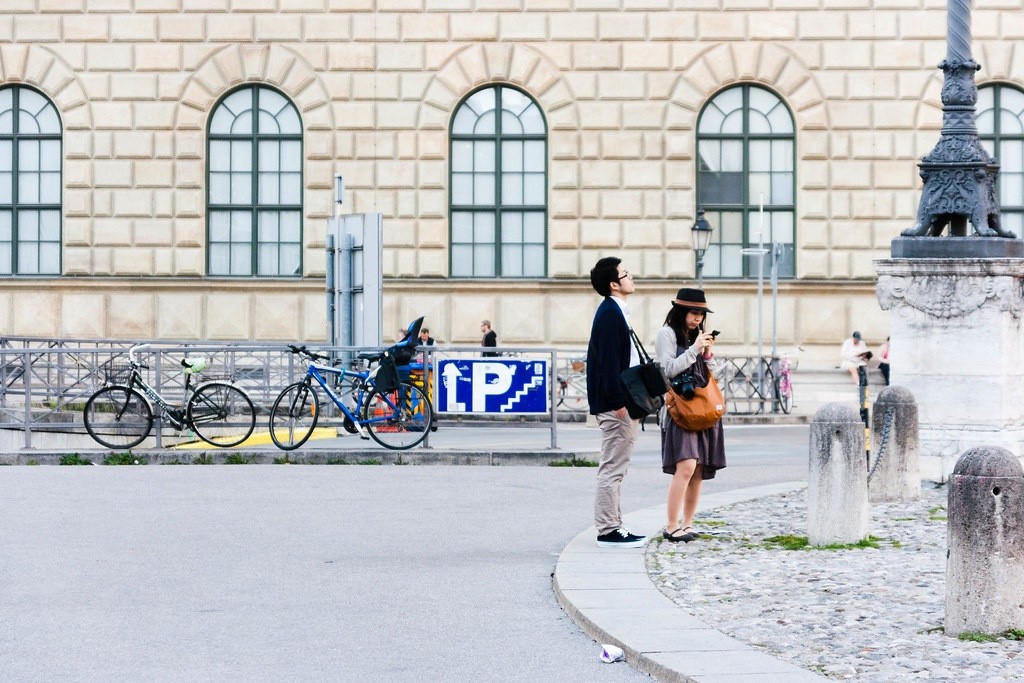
[689,204,713,290]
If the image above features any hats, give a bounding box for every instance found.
[853,331,862,340]
[671,288,714,313]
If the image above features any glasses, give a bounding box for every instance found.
[481,325,486,327]
[612,271,629,283]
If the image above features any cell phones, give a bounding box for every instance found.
[706,330,720,341]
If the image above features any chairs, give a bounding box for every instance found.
[373,316,424,393]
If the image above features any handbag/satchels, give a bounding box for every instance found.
[665,366,726,432]
[618,362,666,419]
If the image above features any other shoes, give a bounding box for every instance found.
[683,527,700,538]
[663,528,694,543]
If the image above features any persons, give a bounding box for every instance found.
[416,327,437,363]
[655,288,727,543]
[480,320,498,357]
[840,330,868,384]
[585,256,648,548]
[876,336,890,386]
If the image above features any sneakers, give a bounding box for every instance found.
[597,527,648,548]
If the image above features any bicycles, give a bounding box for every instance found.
[83,344,257,449]
[777,346,806,414]
[268,316,434,450]
